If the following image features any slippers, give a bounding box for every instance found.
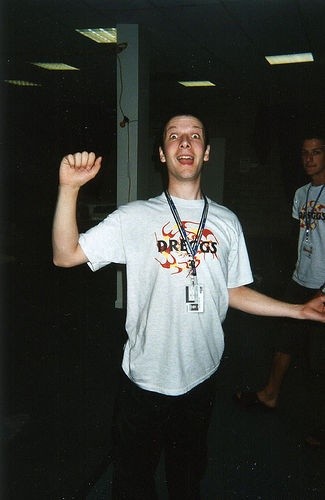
[304,428,325,449]
[233,385,279,413]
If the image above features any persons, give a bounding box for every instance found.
[231,137,325,448]
[51,114,325,500]
